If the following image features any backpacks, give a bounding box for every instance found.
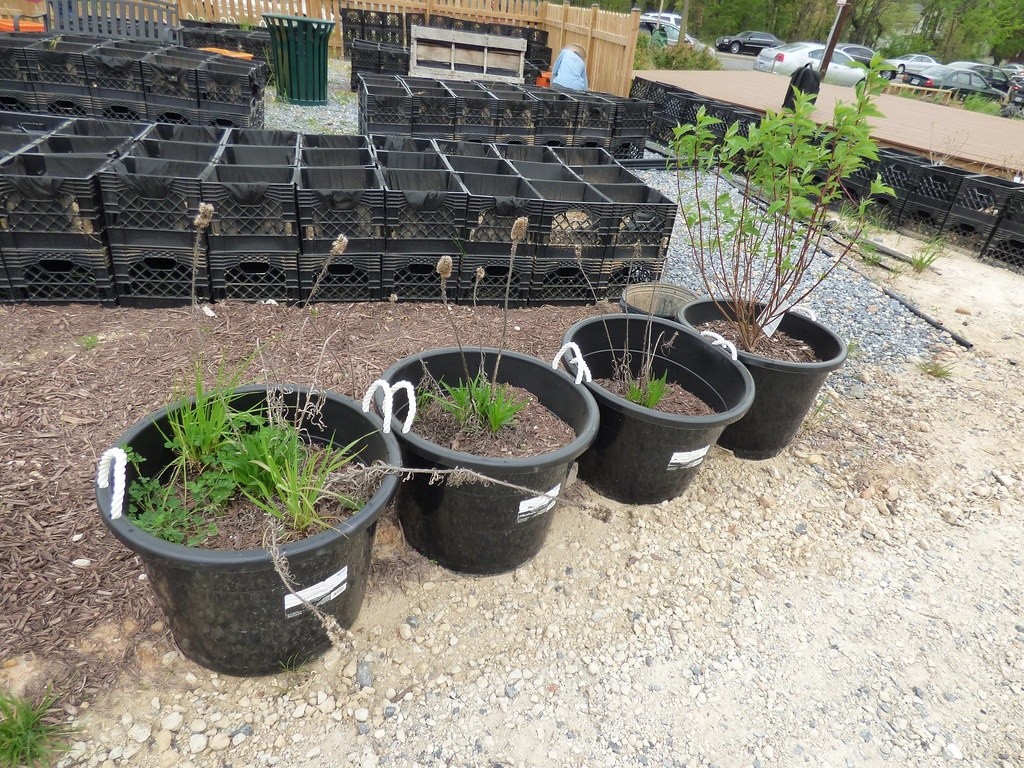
[782,63,819,114]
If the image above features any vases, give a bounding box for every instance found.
[618,280,700,322]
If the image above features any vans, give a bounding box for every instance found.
[641,13,682,29]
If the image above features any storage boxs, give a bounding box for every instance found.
[0,10,1024,305]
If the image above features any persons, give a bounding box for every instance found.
[552,43,588,94]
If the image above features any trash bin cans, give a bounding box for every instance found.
[260,12,337,107]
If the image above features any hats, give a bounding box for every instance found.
[565,43,587,61]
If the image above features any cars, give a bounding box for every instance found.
[753,41,868,87]
[947,60,1024,119]
[884,54,941,75]
[902,64,1008,112]
[835,42,897,81]
[639,18,716,57]
[715,31,787,57]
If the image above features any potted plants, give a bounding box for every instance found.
[563,232,755,506]
[94,330,402,677]
[374,216,601,576]
[669,46,898,462]
